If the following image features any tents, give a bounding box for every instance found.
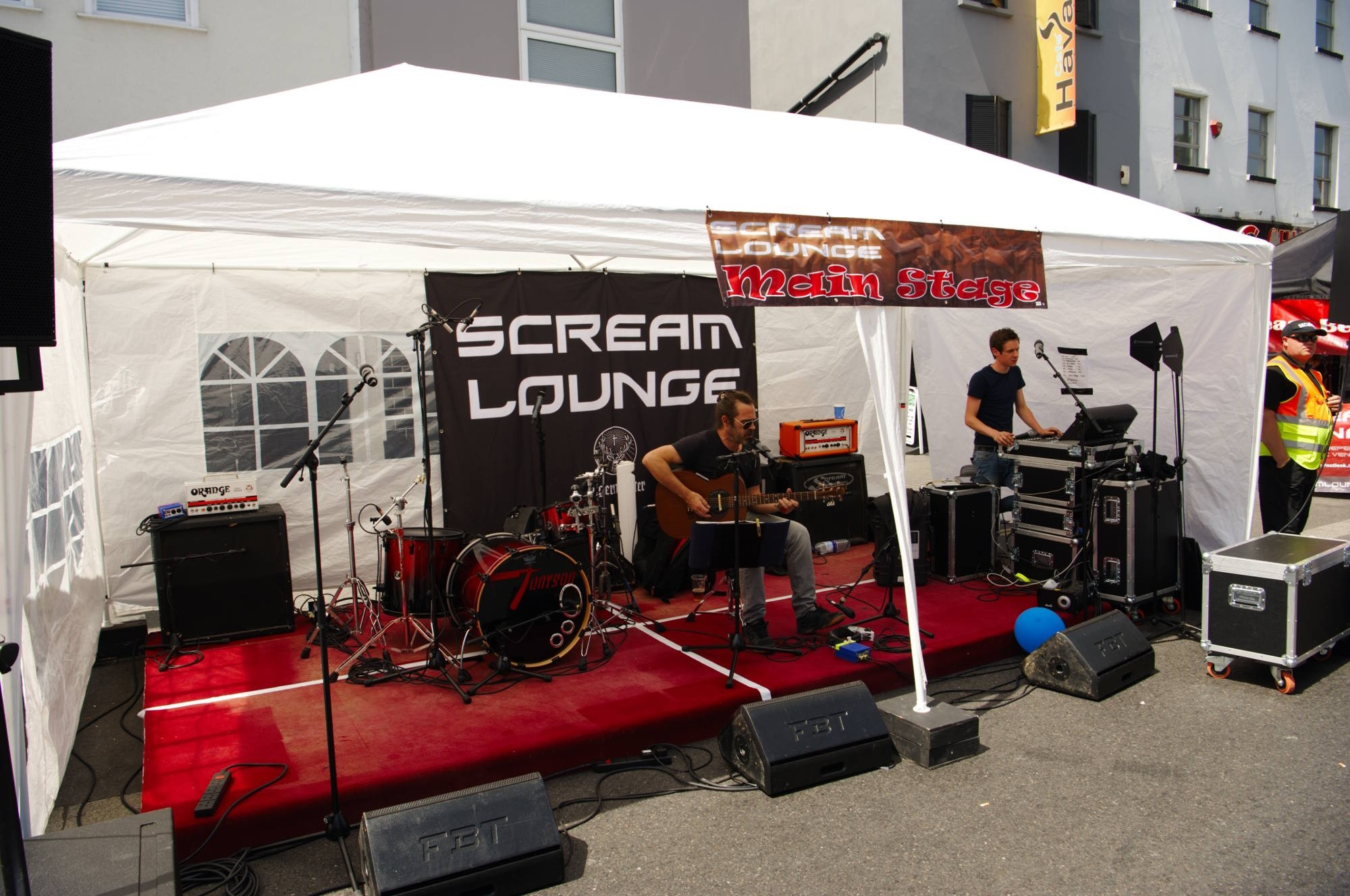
[7,51,1279,836]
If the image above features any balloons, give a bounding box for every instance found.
[1014,608,1065,655]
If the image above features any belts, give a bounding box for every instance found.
[974,446,999,452]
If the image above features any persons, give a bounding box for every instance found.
[1259,321,1344,531]
[641,390,845,654]
[964,327,1062,492]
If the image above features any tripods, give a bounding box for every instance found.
[121,548,246,672]
[681,448,801,688]
[245,371,665,896]
[839,533,934,640]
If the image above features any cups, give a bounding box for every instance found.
[833,404,845,420]
[691,574,706,602]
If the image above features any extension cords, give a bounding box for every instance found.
[193,770,230,818]
[591,753,673,772]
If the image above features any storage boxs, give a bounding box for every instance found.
[1200,530,1350,694]
[921,436,1181,624]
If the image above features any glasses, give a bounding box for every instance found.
[733,417,759,430]
[1288,334,1317,343]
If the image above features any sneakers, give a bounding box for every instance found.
[796,606,846,634]
[740,618,776,652]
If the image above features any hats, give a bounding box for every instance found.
[1282,321,1326,336]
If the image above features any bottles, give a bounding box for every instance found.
[811,538,850,556]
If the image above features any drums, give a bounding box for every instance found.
[540,498,608,546]
[446,532,591,668]
[380,527,466,619]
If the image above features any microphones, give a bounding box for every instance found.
[563,599,581,609]
[1014,429,1038,439]
[460,304,481,332]
[749,437,781,465]
[427,306,453,334]
[375,506,392,525]
[360,364,377,387]
[827,599,856,619]
[530,387,546,425]
[1034,340,1044,359]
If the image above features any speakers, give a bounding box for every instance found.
[151,502,295,651]
[771,455,872,546]
[0,26,56,347]
[358,772,567,896]
[717,681,893,797]
[1020,609,1155,701]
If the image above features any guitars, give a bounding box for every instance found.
[654,468,848,540]
